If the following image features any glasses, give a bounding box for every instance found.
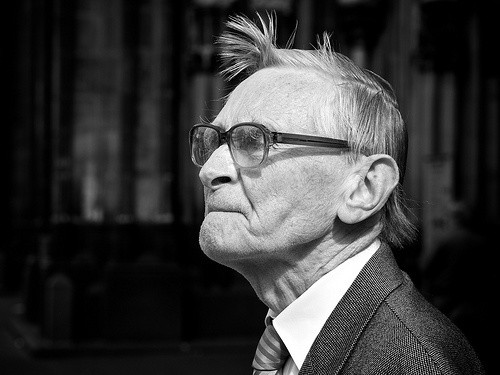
[188,124,372,173]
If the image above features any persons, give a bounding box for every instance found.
[184,8,487,375]
